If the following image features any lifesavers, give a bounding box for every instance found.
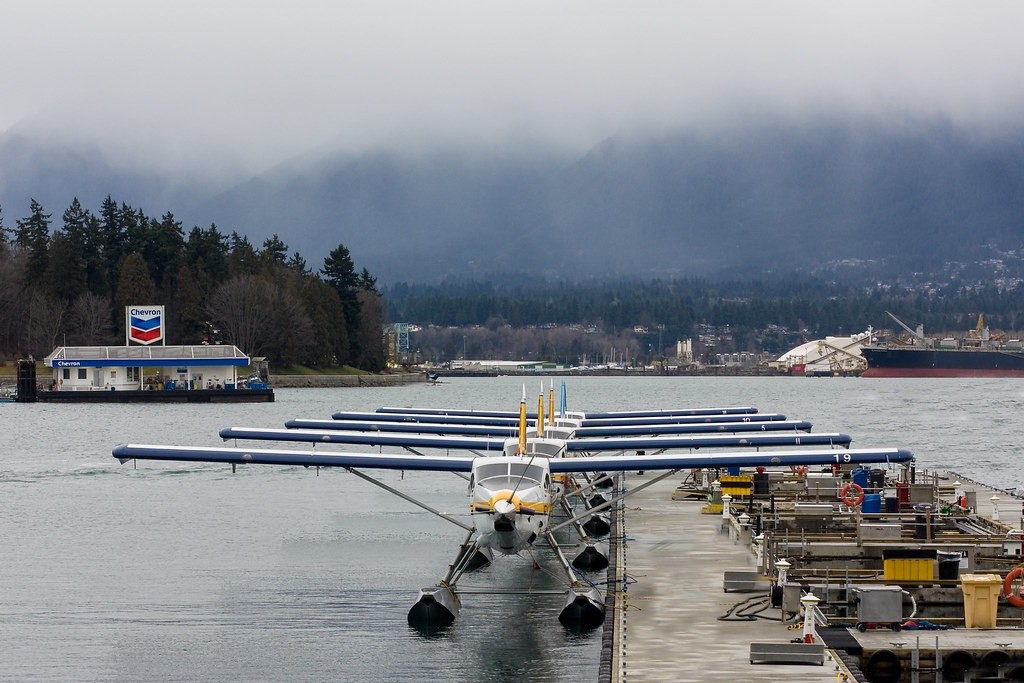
[789,464,808,473]
[841,484,864,507]
[755,475,768,488]
[52,384,57,391]
[1003,568,1024,608]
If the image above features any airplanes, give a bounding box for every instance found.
[111,378,917,636]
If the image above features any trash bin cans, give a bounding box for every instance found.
[739,524,752,545]
[852,468,887,494]
[960,574,1004,630]
[782,583,801,624]
[937,551,961,589]
[914,502,936,539]
[713,490,723,504]
